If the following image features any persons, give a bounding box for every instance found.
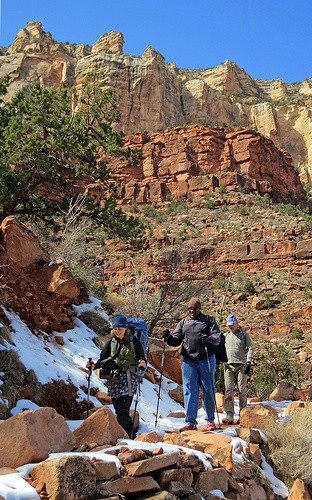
[85,315,147,436]
[161,298,223,431]
[224,313,254,425]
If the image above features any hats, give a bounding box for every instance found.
[111,314,129,328]
[187,296,202,310]
[226,313,236,326]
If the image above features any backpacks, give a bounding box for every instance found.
[206,312,229,364]
[112,317,148,385]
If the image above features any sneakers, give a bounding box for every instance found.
[222,415,235,424]
[206,423,216,431]
[178,424,197,433]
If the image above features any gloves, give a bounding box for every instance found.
[201,334,211,347]
[162,327,171,340]
[242,361,251,374]
